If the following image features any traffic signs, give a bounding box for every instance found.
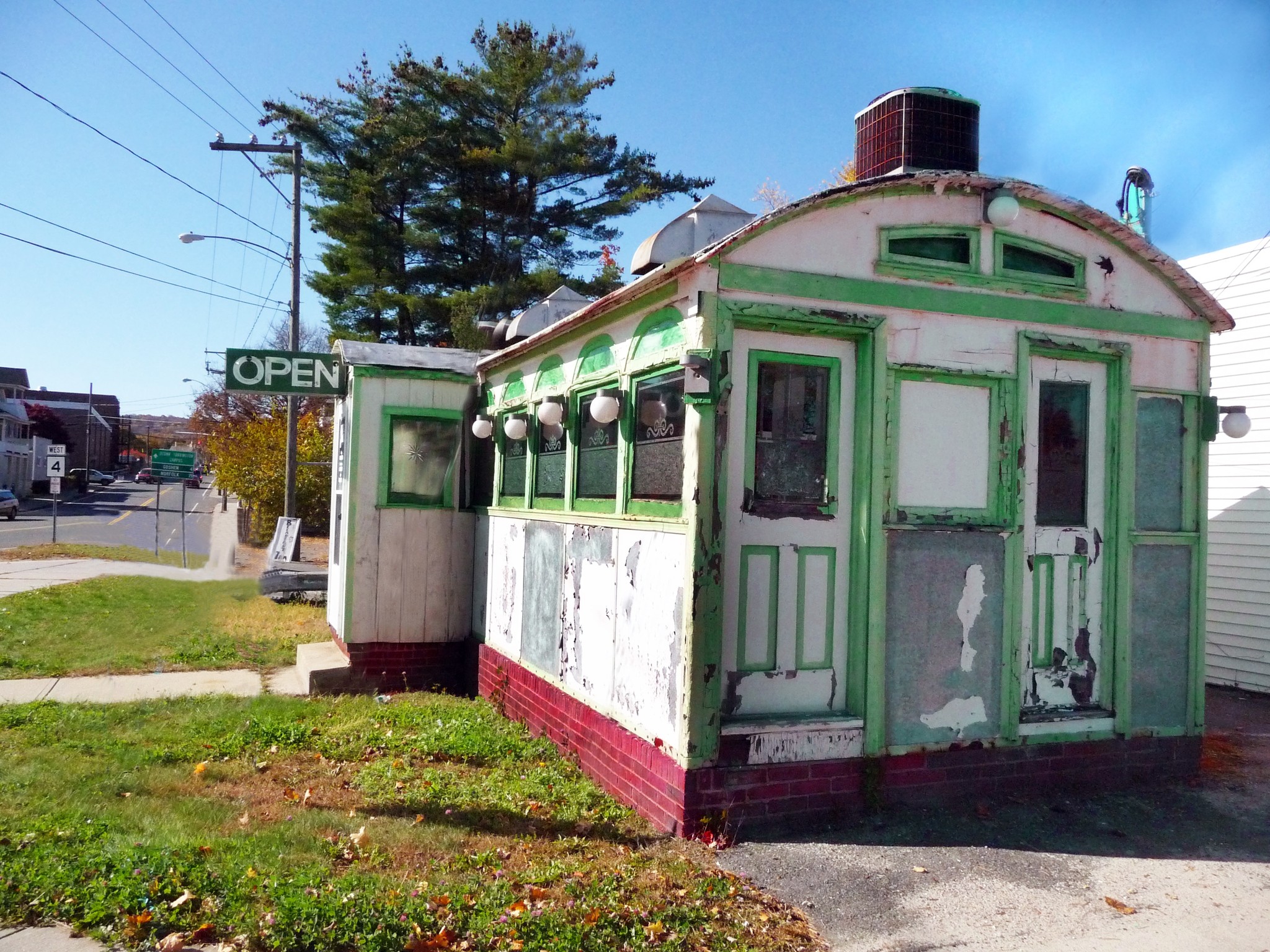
[151,449,196,465]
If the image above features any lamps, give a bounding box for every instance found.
[982,188,1019,227]
[472,415,495,438]
[1219,406,1251,438]
[590,389,623,423]
[504,413,531,439]
[538,396,569,426]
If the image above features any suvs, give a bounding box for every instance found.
[195,466,207,475]
[67,468,116,486]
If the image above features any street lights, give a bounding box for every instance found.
[179,230,300,518]
[145,426,150,467]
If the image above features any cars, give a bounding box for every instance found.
[0,489,19,520]
[193,470,204,483]
[183,475,201,489]
[134,468,163,484]
